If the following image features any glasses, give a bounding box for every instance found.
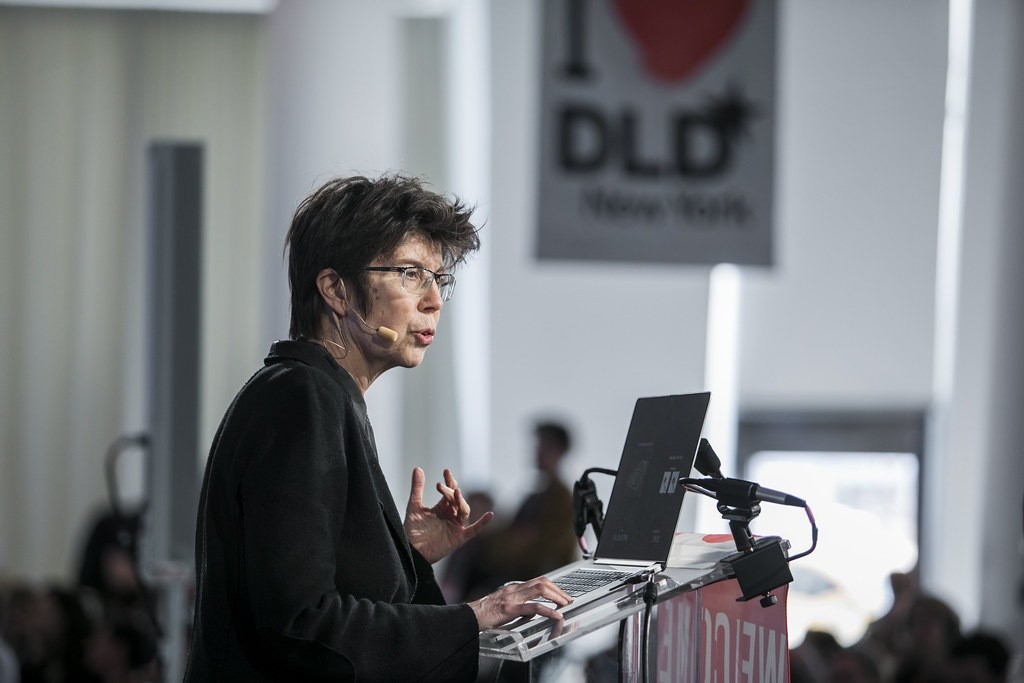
[352,266,456,302]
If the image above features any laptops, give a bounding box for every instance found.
[495,391,712,632]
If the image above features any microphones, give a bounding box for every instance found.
[694,436,723,480]
[340,278,398,341]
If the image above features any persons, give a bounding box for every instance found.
[184,178,574,683]
[788,557,1008,683]
[442,488,496,604]
[0,510,165,683]
[490,421,580,587]
